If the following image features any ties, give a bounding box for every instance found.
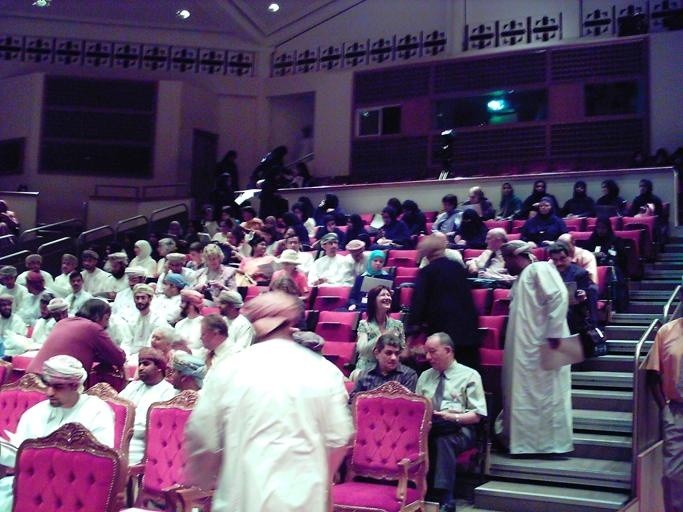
[483,252,494,271]
[431,374,445,410]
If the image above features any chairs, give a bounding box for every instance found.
[465,274,513,427]
[337,250,351,256]
[596,266,619,325]
[609,201,668,281]
[483,218,527,240]
[400,333,427,362]
[458,249,485,263]
[383,249,420,313]
[456,391,497,482]
[1,356,220,511]
[416,212,437,243]
[201,307,221,316]
[309,227,319,246]
[336,226,348,232]
[362,311,406,323]
[245,278,270,302]
[331,380,433,510]
[360,213,376,245]
[186,254,192,261]
[562,217,598,247]
[304,286,361,378]
[531,246,550,261]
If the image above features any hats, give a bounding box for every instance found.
[345,239,366,251]
[275,249,301,265]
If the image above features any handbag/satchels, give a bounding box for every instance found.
[430,413,459,438]
